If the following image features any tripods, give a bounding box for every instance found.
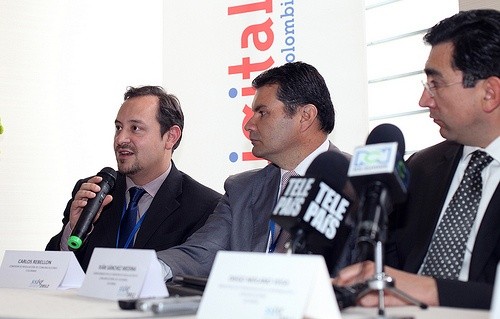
[340,228,430,318]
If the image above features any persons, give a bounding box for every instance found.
[45,86,223,273]
[157,62,353,278]
[333,10,500,311]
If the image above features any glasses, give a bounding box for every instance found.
[422,74,476,98]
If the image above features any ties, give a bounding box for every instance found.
[273,171,301,253]
[423,150,494,280]
[118,187,147,248]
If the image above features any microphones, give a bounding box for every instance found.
[347,123,410,260]
[271,150,353,258]
[68,167,117,249]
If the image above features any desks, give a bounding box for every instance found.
[0,286,491,319]
